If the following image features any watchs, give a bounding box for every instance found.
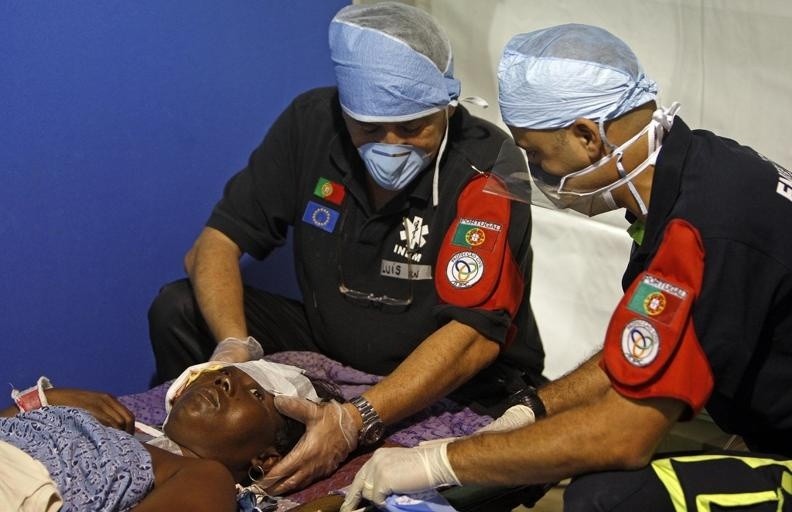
[350,395,386,446]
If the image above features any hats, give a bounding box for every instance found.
[328,2,462,121]
[498,24,657,129]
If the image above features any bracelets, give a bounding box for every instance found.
[8,375,53,412]
[504,385,550,422]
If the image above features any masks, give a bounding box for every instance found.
[521,151,619,220]
[353,142,436,192]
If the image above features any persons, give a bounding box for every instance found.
[145,0,552,499]
[1,358,347,511]
[329,22,792,512]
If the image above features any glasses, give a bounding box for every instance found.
[338,209,414,307]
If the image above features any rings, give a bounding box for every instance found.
[363,480,375,490]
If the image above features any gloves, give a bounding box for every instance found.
[339,443,462,512]
[416,406,538,446]
